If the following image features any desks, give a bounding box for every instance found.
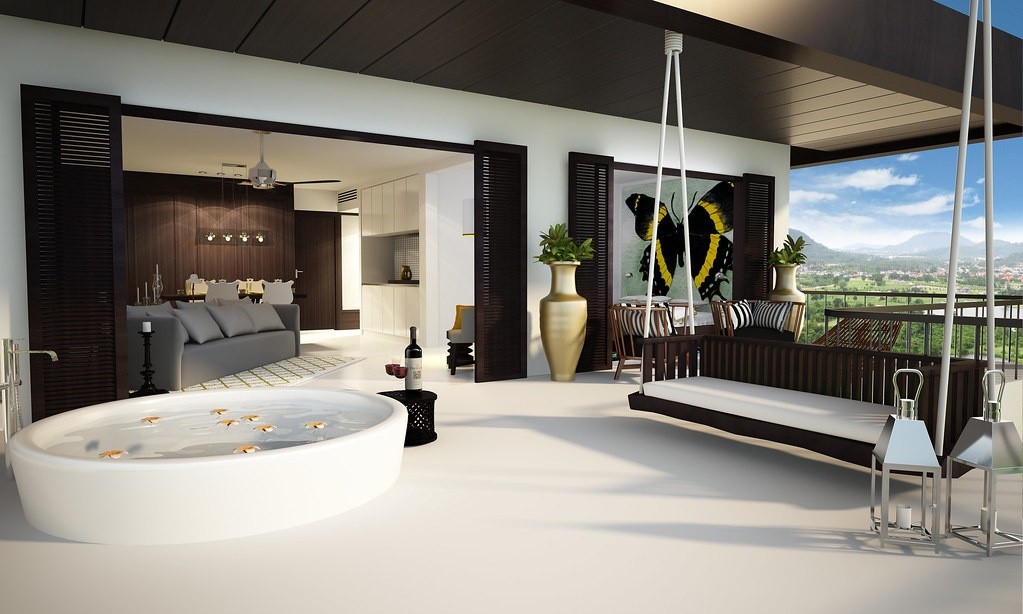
[159,294,307,308]
[380,389,438,447]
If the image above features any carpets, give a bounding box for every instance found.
[180,355,368,392]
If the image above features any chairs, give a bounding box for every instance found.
[447,305,475,375]
[184,274,294,303]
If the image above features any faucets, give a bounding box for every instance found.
[2,338,59,383]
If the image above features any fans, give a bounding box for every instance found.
[185,131,342,190]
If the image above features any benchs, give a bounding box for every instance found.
[711,299,806,343]
[609,303,696,380]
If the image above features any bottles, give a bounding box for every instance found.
[405,326,422,394]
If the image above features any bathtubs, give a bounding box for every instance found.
[8,386,409,547]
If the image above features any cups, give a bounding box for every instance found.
[385,364,400,374]
[394,366,406,378]
[981,506,997,531]
[896,504,912,529]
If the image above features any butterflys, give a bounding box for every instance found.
[625,180,734,304]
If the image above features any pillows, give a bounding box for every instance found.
[619,302,679,337]
[127,297,286,345]
[752,300,793,332]
[716,299,754,329]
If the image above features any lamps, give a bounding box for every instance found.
[205,232,265,242]
[249,162,277,189]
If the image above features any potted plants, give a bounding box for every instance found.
[766,236,811,343]
[533,224,598,382]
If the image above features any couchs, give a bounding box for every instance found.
[127,304,300,390]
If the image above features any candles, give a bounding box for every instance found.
[896,503,912,528]
[143,322,151,332]
[136,264,160,302]
[980,507,996,531]
